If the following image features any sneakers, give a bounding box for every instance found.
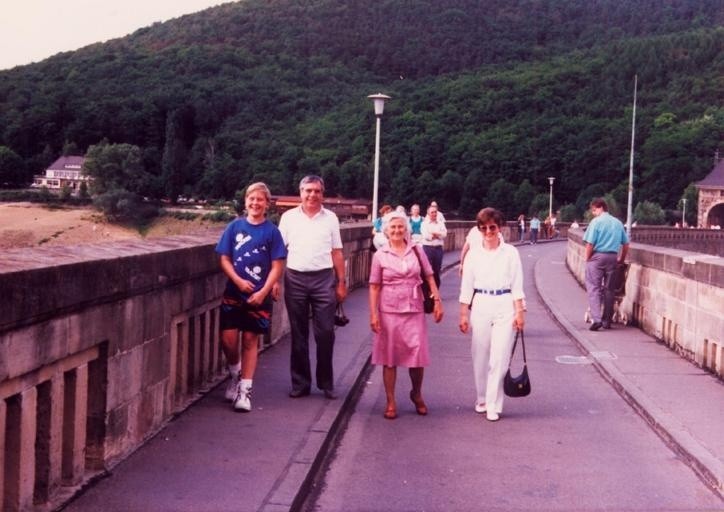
[225,370,252,411]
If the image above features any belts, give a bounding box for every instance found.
[475,289,510,294]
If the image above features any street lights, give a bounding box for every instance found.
[547,176,556,218]
[682,198,687,227]
[367,92,392,221]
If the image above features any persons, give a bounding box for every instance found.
[460,225,504,274]
[271,175,347,399]
[458,207,525,421]
[370,201,448,297]
[582,199,630,330]
[369,212,444,419]
[517,213,557,245]
[214,182,287,410]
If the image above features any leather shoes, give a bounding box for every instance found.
[289,388,310,397]
[589,320,610,330]
[326,389,338,398]
[475,404,499,421]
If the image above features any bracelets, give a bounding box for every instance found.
[434,299,442,301]
[337,279,346,283]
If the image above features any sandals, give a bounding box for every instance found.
[411,390,427,414]
[385,405,396,418]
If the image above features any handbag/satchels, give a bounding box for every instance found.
[420,271,440,313]
[504,365,530,397]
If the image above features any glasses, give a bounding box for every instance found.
[480,226,495,231]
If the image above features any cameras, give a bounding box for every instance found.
[333,315,349,327]
